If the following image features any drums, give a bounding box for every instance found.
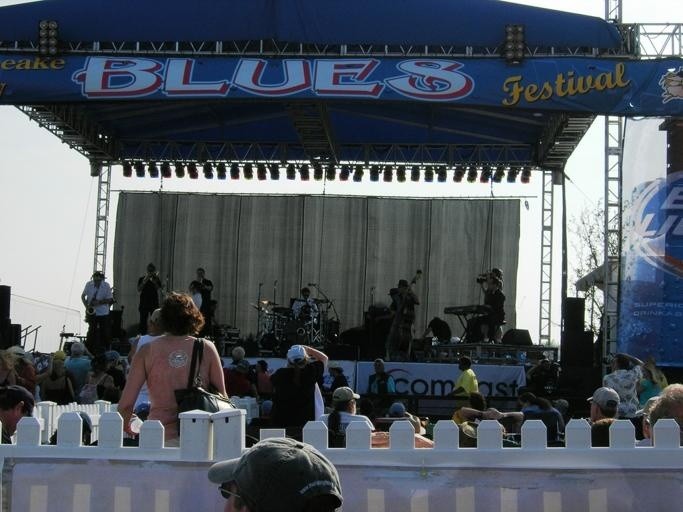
[259,318,339,358]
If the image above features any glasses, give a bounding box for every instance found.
[216,485,240,503]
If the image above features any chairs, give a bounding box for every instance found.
[237,410,563,447]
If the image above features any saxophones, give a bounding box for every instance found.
[84,291,98,322]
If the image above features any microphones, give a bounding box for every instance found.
[417,269,422,274]
[60,333,74,337]
[308,283,316,286]
[259,283,263,286]
[274,280,278,283]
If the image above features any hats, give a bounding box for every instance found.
[206,436,345,510]
[634,396,661,426]
[388,402,406,418]
[0,385,34,417]
[586,386,620,411]
[286,344,312,364]
[331,386,360,402]
[386,279,408,295]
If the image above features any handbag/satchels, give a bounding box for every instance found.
[173,386,236,436]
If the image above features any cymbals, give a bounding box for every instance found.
[257,300,279,305]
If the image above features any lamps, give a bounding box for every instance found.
[119,159,533,183]
[38,20,61,59]
[504,23,525,64]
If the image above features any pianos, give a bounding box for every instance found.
[444,304,491,314]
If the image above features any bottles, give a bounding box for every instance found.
[128,413,144,434]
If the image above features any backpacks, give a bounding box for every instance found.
[78,383,99,404]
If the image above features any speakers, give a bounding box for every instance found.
[0,285,21,350]
[502,329,532,345]
[561,298,593,397]
